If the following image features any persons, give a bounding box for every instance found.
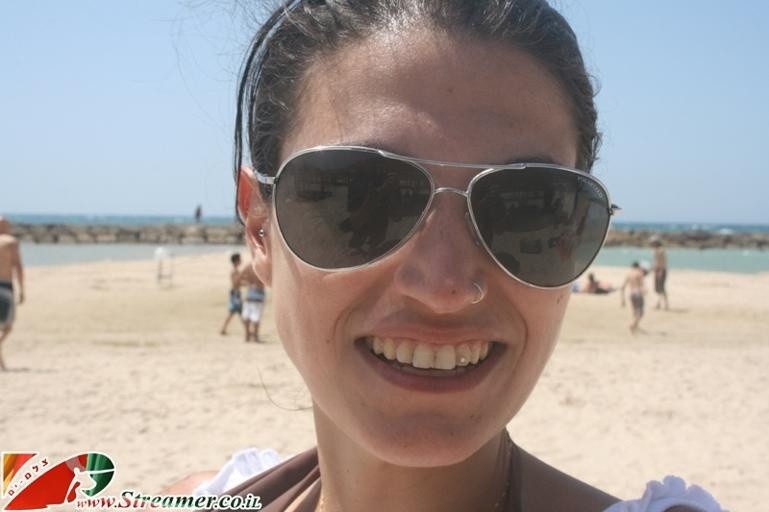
[336,152,405,261]
[643,235,673,314]
[180,0,715,512]
[619,260,646,336]
[571,272,602,295]
[1,217,26,368]
[238,264,266,342]
[219,252,242,339]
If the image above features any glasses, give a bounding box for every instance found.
[255,144,621,289]
